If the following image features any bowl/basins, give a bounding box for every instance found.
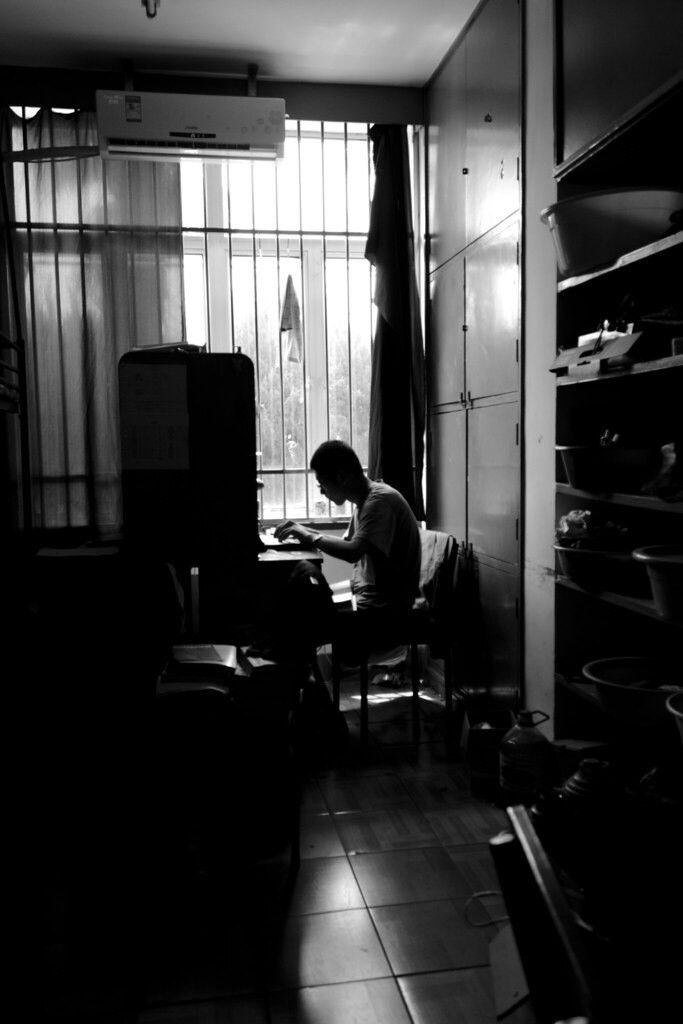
[554,541,641,590]
[633,545,683,624]
[665,689,683,741]
[582,656,683,727]
[555,443,642,493]
[542,186,683,276]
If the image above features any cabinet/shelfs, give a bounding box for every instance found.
[427,0,683,745]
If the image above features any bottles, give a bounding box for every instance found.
[563,757,610,828]
[500,711,553,805]
[528,790,569,852]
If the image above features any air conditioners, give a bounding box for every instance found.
[96,90,286,161]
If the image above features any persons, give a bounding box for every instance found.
[273,441,423,649]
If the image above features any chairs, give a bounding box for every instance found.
[333,529,457,730]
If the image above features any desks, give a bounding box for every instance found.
[258,549,323,571]
